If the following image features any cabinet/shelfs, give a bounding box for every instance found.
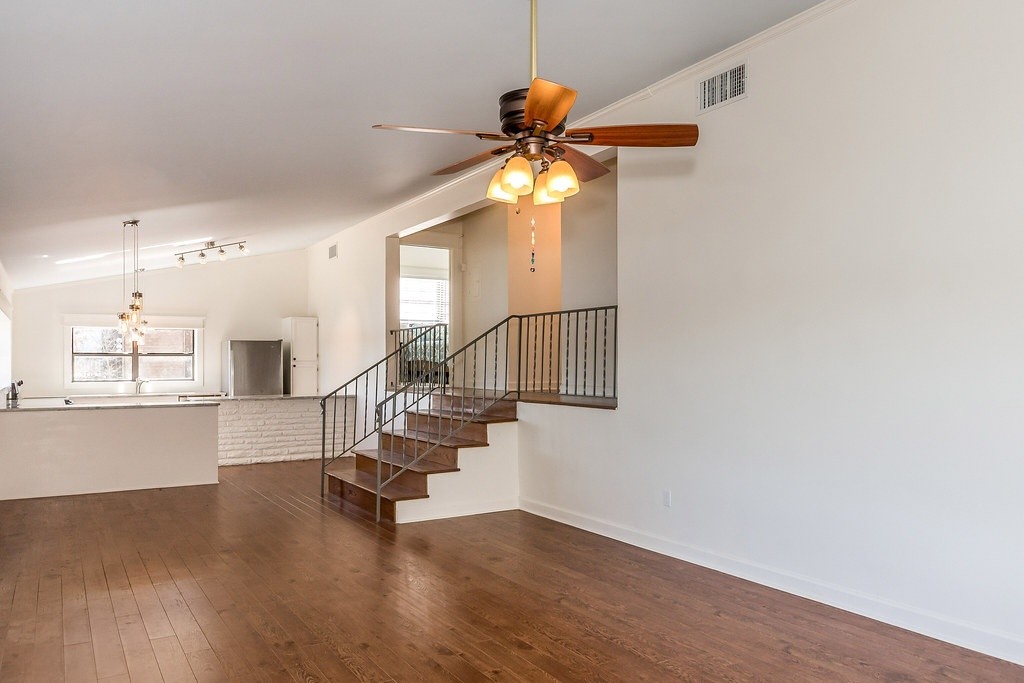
[280,316,318,395]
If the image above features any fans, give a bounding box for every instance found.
[372,0,699,183]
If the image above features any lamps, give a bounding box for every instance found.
[500,150,535,196]
[174,241,249,267]
[116,219,143,335]
[542,147,581,198]
[532,158,564,207]
[486,164,519,205]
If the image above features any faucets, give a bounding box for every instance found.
[135,378,150,395]
